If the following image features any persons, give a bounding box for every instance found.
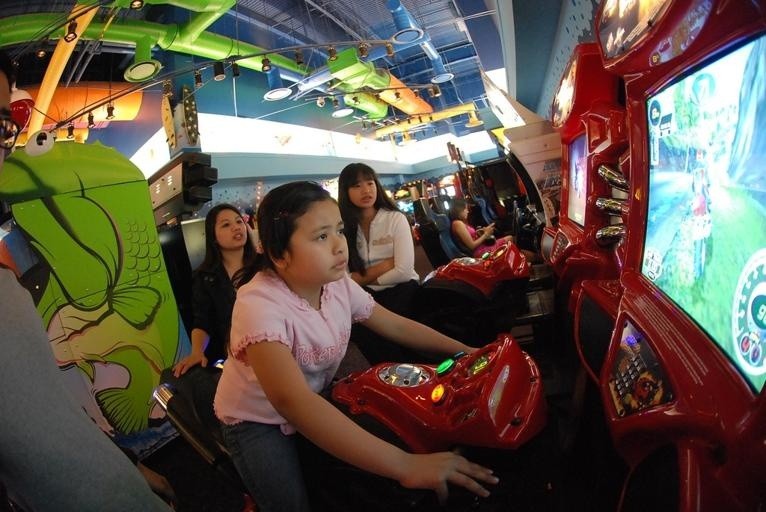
[449,195,543,262]
[170,203,272,378]
[214,180,500,512]
[334,162,449,367]
[691,149,712,206]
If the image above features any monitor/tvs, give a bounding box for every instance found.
[566,131,587,230]
[636,18,766,405]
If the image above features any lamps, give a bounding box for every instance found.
[361,114,434,131]
[191,53,240,88]
[357,42,395,59]
[66,102,116,139]
[34,16,78,60]
[332,82,443,109]
[262,45,340,72]
